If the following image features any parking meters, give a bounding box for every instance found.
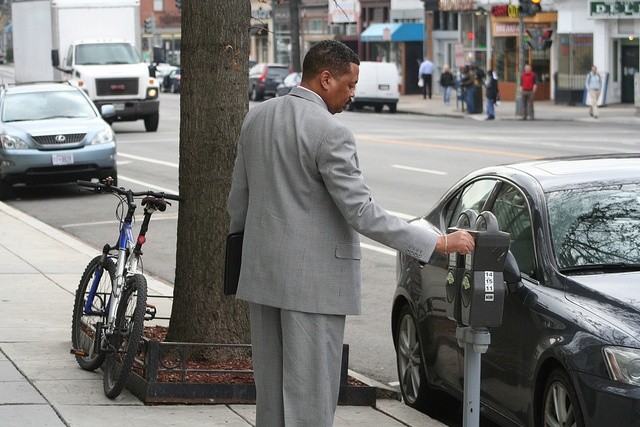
[445,207,511,427]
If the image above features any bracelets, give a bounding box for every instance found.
[444,234,448,256]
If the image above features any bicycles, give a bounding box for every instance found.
[69,176,187,400]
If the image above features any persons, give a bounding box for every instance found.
[455,64,470,113]
[483,71,498,120]
[465,64,474,81]
[519,64,538,120]
[439,64,454,104]
[417,56,436,99]
[148,58,162,78]
[585,65,602,119]
[471,66,487,87]
[226,39,475,425]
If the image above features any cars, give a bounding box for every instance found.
[344,61,400,113]
[248,63,294,101]
[0,79,118,201]
[163,69,181,93]
[390,152,640,427]
[275,72,303,97]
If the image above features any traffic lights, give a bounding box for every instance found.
[518,0,541,17]
[144,16,154,35]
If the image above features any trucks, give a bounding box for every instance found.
[9,2,166,131]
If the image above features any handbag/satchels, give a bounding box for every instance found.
[223,232,244,295]
